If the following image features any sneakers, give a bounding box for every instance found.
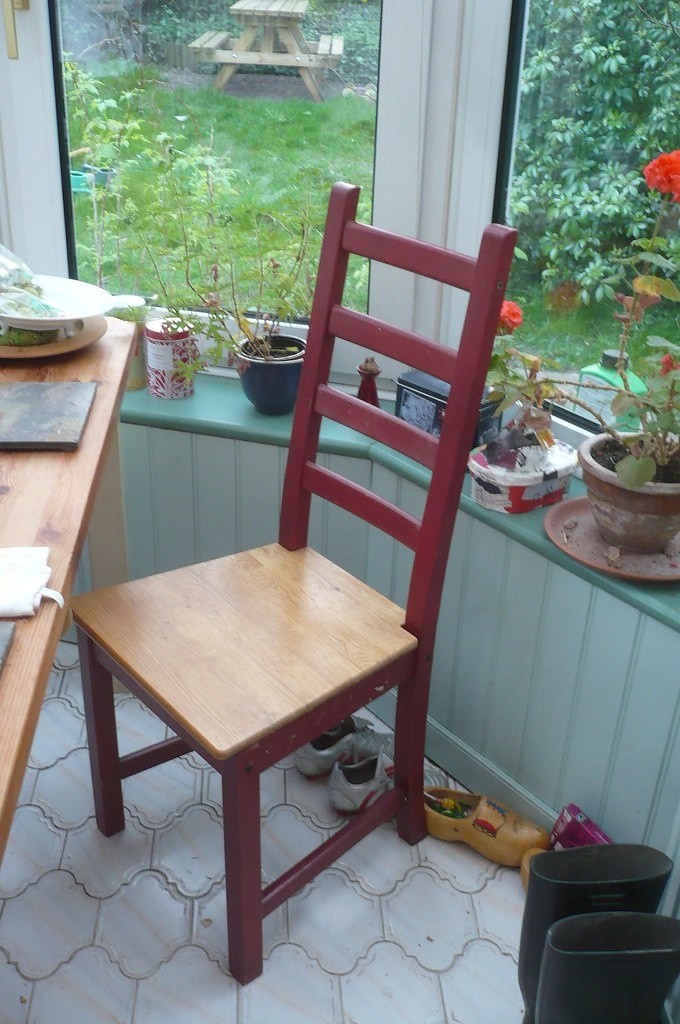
[296,715,395,779]
[331,754,454,813]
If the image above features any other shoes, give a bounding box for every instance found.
[521,851,546,888]
[423,786,551,865]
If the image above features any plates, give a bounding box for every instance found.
[0,273,116,332]
[0,317,108,359]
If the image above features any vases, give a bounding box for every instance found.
[577,432,680,555]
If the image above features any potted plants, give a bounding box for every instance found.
[160,174,317,417]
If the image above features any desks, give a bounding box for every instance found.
[0,321,136,863]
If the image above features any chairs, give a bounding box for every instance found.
[71,180,517,985]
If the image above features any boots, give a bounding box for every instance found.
[533,912,680,1024]
[516,843,672,1023]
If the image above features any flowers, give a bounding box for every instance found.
[532,149,680,487]
[484,300,578,470]
